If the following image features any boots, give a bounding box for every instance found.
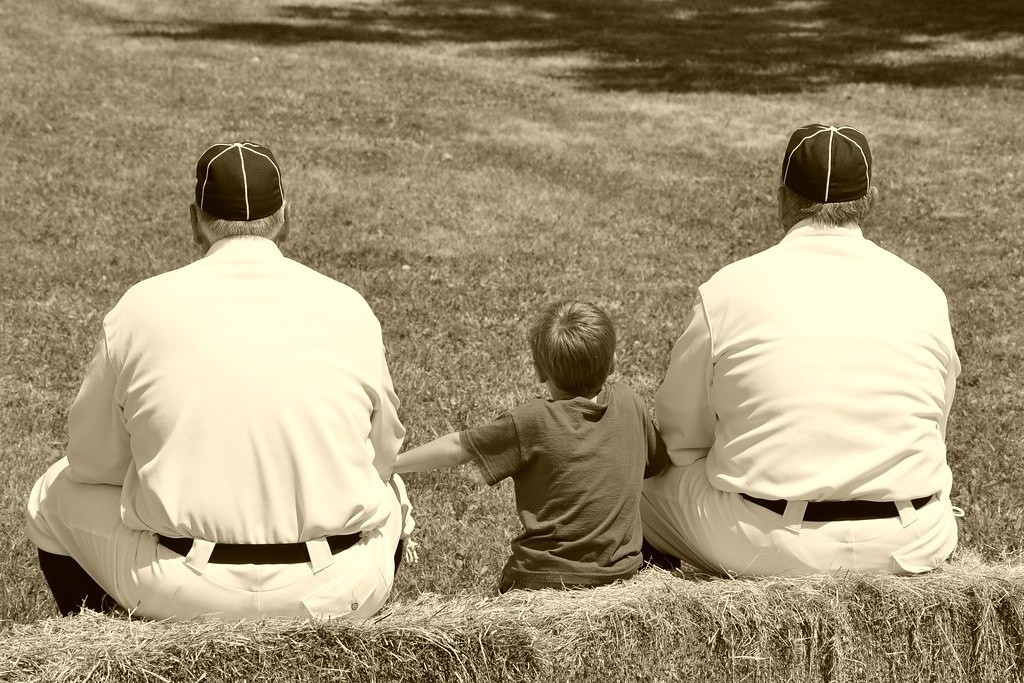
[38,547,117,618]
[637,532,682,572]
[394,539,406,576]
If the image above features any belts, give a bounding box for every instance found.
[740,487,933,522]
[156,531,362,565]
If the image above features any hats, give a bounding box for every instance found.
[782,123,873,203]
[195,142,285,222]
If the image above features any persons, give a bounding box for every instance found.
[23,141,417,623]
[393,301,667,597]
[641,123,961,582]
